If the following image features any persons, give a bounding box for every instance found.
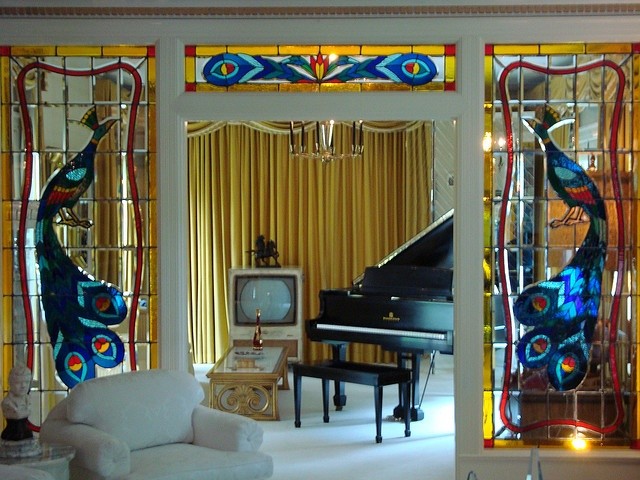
[2,362,32,420]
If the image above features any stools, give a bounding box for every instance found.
[292,361,410,443]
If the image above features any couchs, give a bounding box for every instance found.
[39,369,273,480]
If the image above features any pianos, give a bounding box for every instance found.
[317,323,447,340]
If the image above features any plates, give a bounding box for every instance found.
[235,351,264,359]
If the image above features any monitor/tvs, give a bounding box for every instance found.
[229,264,302,338]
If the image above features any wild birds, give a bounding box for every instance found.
[34,104,127,390]
[513,103,608,392]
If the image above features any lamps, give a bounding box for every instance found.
[288,120,364,163]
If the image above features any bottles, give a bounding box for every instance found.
[253,309,264,350]
